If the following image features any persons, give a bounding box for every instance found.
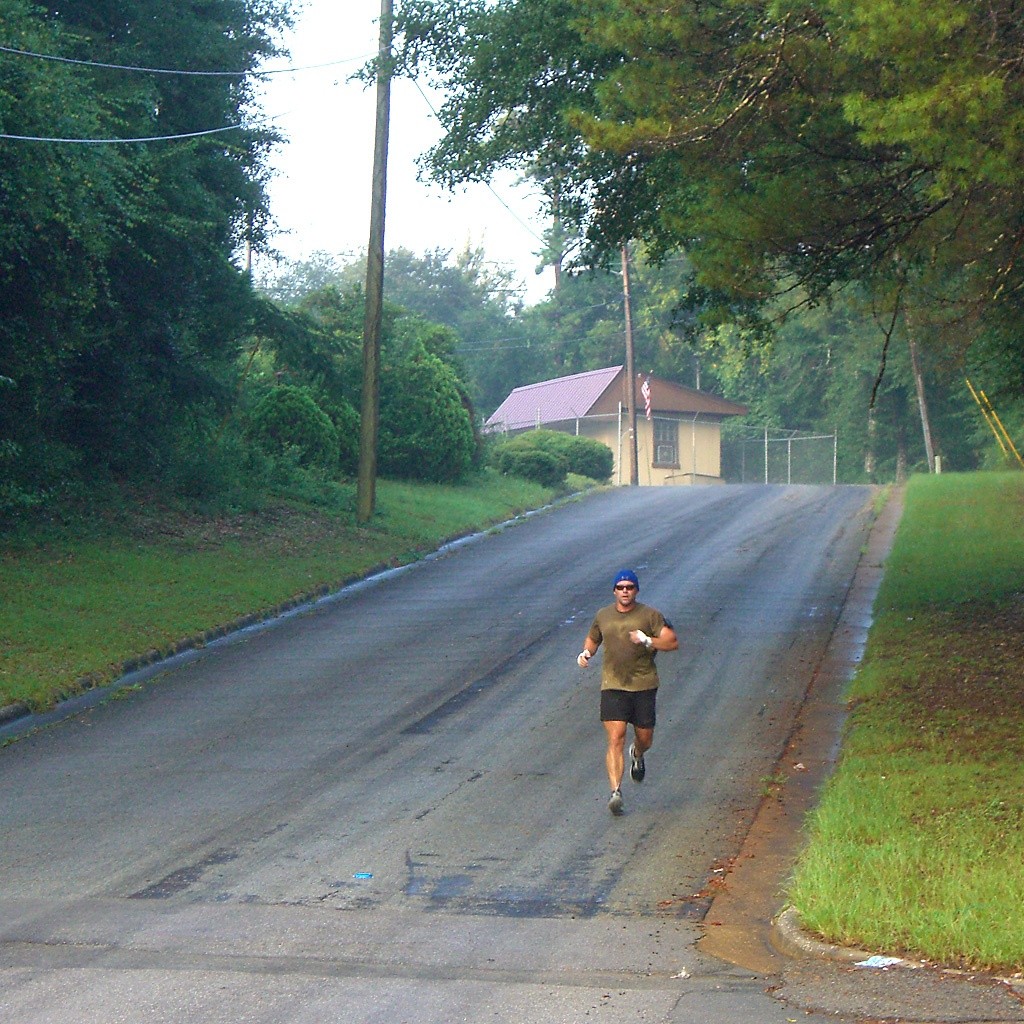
[576,569,680,816]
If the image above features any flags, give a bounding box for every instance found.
[641,372,652,421]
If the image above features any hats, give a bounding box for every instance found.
[612,570,639,591]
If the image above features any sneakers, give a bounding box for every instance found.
[629,743,645,783]
[608,789,624,814]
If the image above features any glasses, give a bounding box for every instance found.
[616,585,636,590]
